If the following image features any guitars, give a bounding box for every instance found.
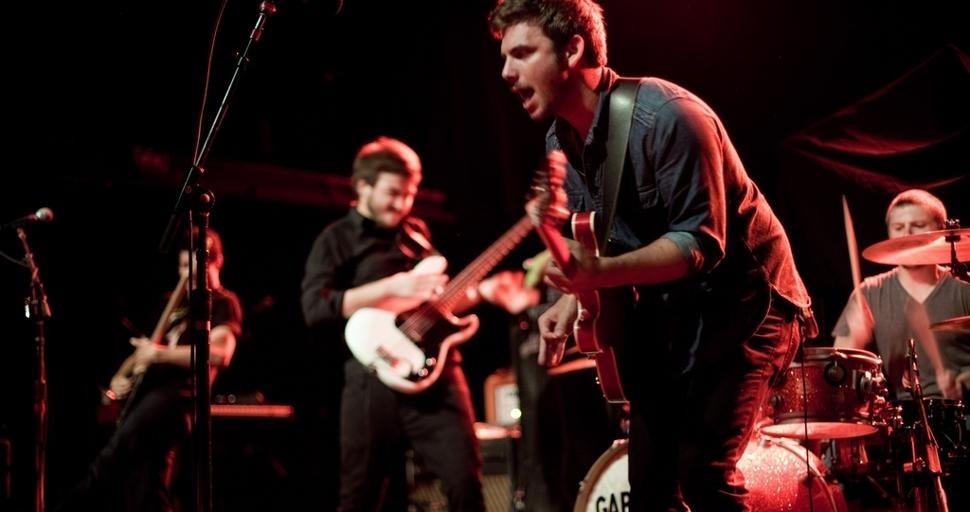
[344,215,535,394]
[525,149,629,405]
[116,236,213,428]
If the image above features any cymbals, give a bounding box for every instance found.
[863,229,969,266]
[928,316,969,335]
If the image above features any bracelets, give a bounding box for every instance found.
[156,344,166,363]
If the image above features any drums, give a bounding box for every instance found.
[896,396,970,479]
[761,346,889,439]
[573,429,898,512]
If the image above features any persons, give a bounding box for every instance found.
[510,246,578,512]
[487,0,819,511]
[298,133,542,508]
[47,223,247,509]
[833,186,969,509]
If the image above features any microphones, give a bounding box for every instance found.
[0,207,53,229]
[901,338,919,391]
